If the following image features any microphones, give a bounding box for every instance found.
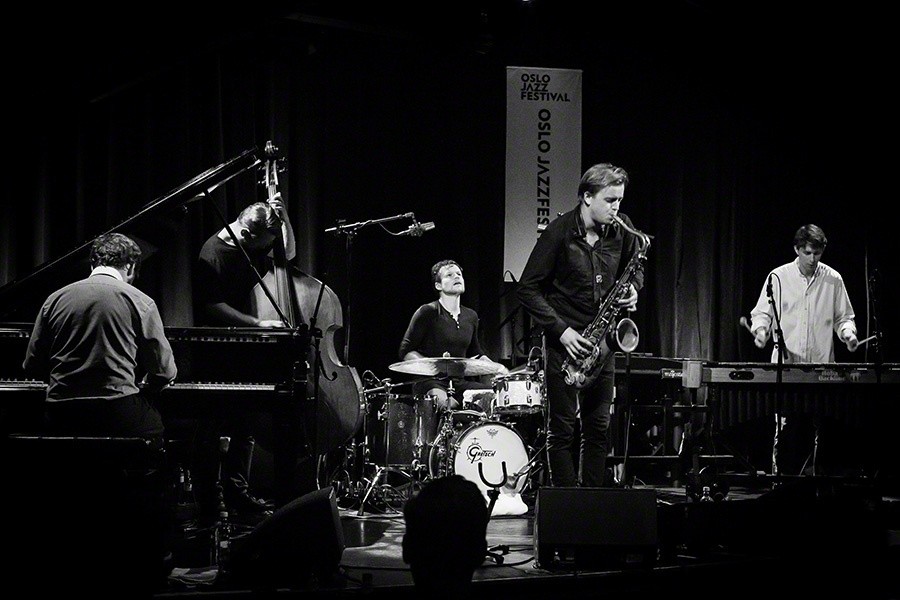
[526,349,533,370]
[509,272,518,288]
[398,222,435,235]
[767,276,772,297]
[368,371,382,387]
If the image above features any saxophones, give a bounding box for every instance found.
[558,218,653,389]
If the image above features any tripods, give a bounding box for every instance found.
[328,367,449,518]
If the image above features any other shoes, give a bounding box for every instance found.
[198,482,225,511]
[222,479,268,511]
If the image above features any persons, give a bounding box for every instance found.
[515,163,645,487]
[22,233,177,504]
[750,223,858,490]
[398,474,490,600]
[398,260,506,468]
[194,191,298,515]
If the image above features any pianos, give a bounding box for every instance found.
[0,145,317,496]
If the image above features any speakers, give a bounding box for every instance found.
[754,481,887,552]
[534,486,660,570]
[229,487,347,585]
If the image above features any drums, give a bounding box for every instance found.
[364,392,443,470]
[494,373,545,417]
[426,419,533,511]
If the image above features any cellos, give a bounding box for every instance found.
[247,137,372,494]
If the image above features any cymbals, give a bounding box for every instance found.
[387,357,506,379]
